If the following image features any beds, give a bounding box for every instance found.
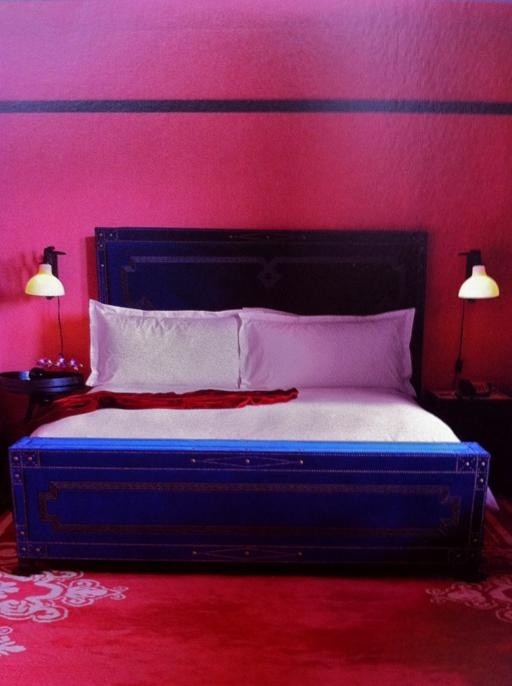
[6,227,491,585]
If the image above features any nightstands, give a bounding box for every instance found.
[429,389,511,501]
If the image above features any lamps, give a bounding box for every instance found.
[20,245,69,302]
[457,246,500,302]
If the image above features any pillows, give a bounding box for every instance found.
[239,307,416,397]
[86,299,241,394]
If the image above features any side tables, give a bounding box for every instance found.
[1,367,84,422]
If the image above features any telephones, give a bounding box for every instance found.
[460,377,489,397]
